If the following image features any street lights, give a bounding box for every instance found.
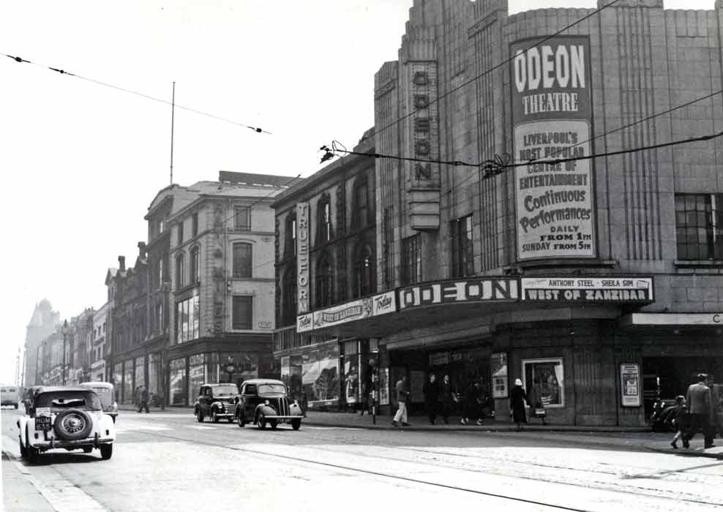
[62,318,69,387]
[369,358,376,424]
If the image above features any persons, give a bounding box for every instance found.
[389,374,411,428]
[422,372,494,425]
[508,376,534,431]
[663,393,690,449]
[136,384,150,414]
[681,372,716,449]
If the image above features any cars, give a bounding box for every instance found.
[194,378,304,429]
[649,394,686,432]
[0,382,118,464]
